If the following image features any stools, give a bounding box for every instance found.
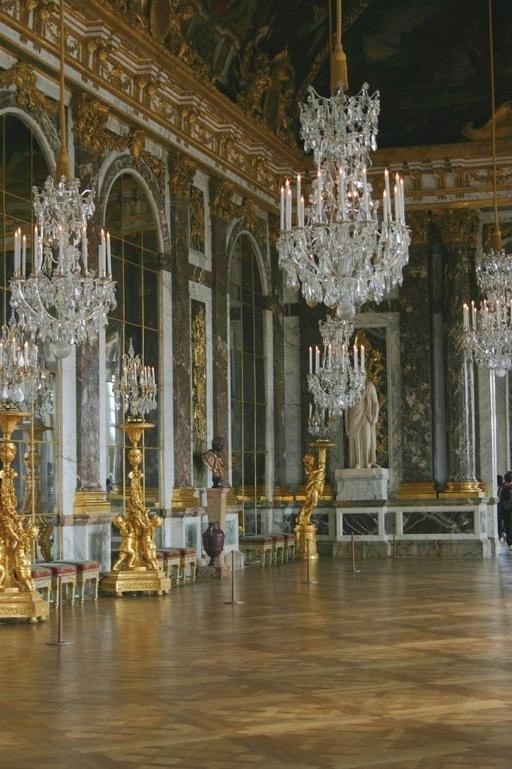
[31,560,100,609]
[155,547,198,584]
[239,534,296,567]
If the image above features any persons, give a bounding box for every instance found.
[110,515,136,571]
[345,368,382,467]
[297,447,326,523]
[497,471,512,551]
[7,517,40,593]
[137,510,162,570]
[202,436,226,483]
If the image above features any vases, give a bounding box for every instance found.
[203,521,224,566]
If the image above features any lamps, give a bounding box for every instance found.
[462,0,512,377]
[306,313,368,417]
[275,0,413,322]
[16,346,54,565]
[100,338,171,597]
[293,395,336,559]
[8,0,119,346]
[0,323,50,624]
